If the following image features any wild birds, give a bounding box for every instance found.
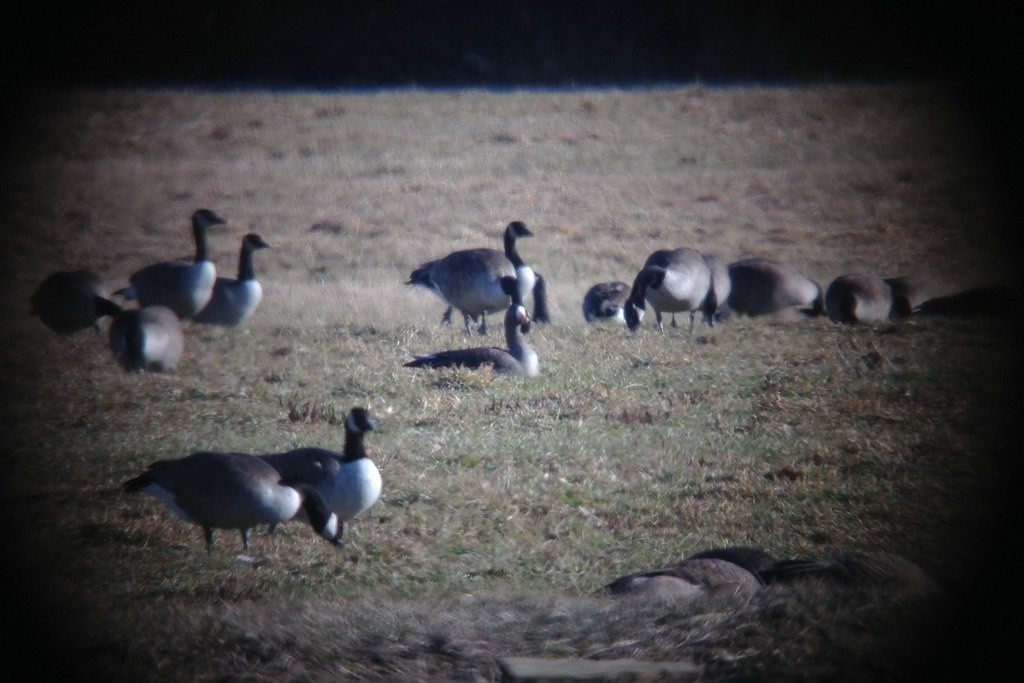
[401,302,539,379]
[106,304,186,380]
[256,406,385,552]
[581,245,1022,337]
[191,232,274,334]
[111,207,232,334]
[121,449,332,557]
[587,546,844,603]
[402,221,551,338]
[25,265,115,341]
